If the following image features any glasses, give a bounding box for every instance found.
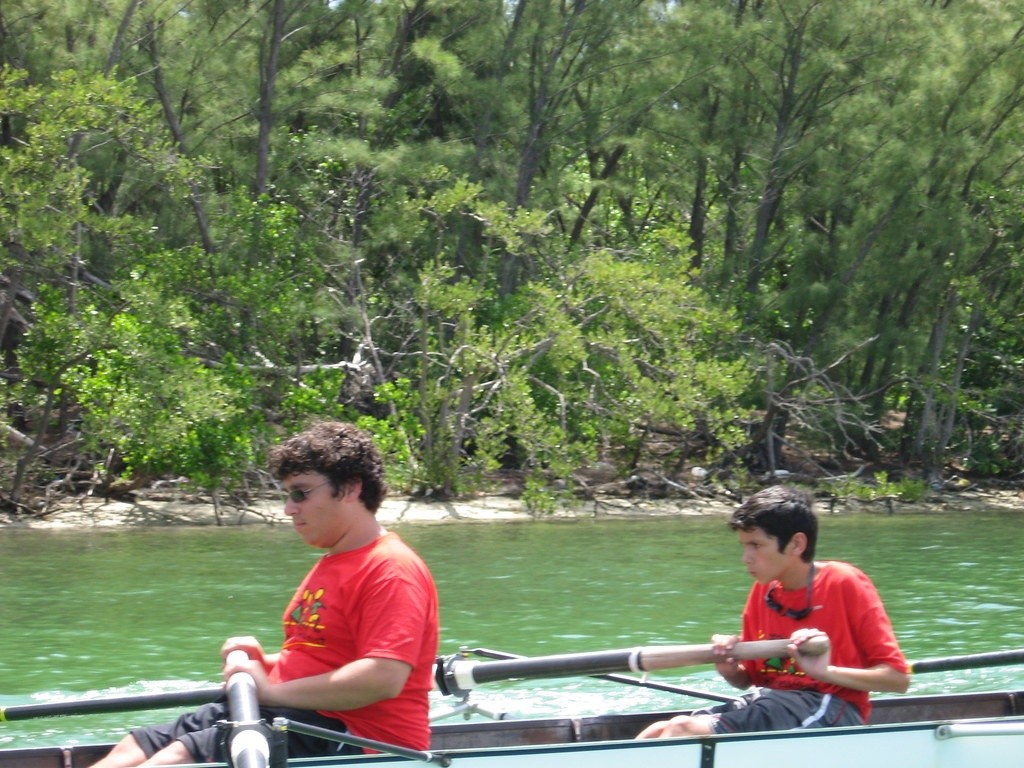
[766,587,812,618]
[281,480,330,503]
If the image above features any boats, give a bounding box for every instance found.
[0,692,1024,768]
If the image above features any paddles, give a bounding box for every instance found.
[0,632,830,724]
[222,650,274,767]
[906,647,1024,676]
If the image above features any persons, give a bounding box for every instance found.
[635,484,913,740]
[87,420,439,768]
